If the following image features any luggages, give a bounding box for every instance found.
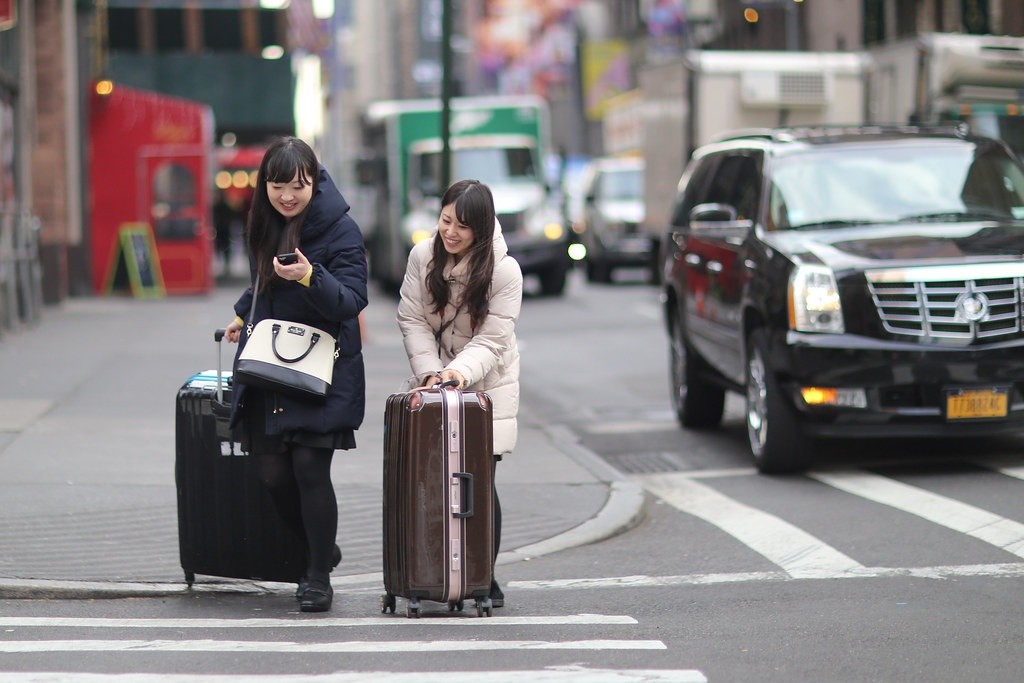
[175,326,308,595]
[380,380,496,618]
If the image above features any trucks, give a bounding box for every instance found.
[865,30,1024,162]
[363,93,572,298]
[641,45,869,284]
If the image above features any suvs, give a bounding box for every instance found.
[659,125,1024,478]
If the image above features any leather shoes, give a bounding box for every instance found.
[300,575,333,612]
[296,575,309,601]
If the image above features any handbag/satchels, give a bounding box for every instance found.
[235,255,345,399]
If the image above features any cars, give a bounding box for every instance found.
[577,157,648,286]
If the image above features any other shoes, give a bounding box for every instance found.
[488,578,505,607]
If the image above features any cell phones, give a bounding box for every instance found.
[278,253,299,265]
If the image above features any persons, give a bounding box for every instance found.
[396,178,526,610]
[224,136,371,613]
